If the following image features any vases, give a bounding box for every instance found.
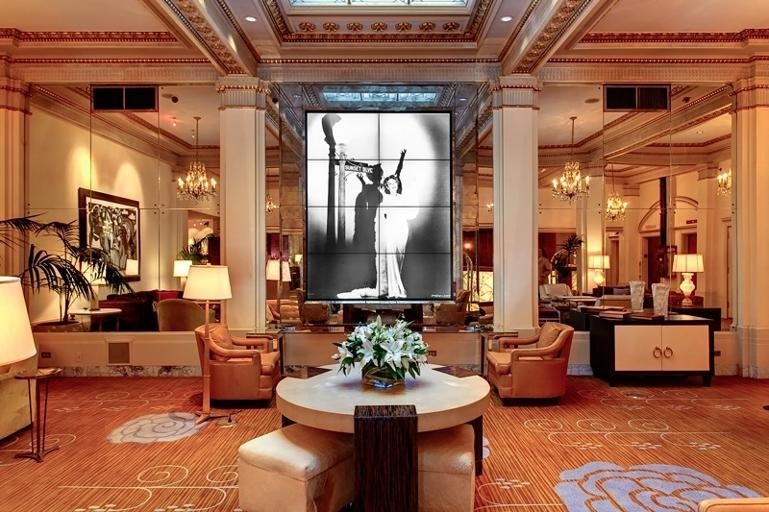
[651,283,671,319]
[627,279,646,312]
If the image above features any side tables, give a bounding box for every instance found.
[479,328,519,374]
[244,327,284,378]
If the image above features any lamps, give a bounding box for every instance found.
[183,265,234,424]
[266,259,293,316]
[0,275,38,369]
[175,117,218,203]
[172,259,192,283]
[551,117,629,223]
[672,253,704,306]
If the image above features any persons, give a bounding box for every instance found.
[355,147,407,249]
[88,202,138,271]
[372,173,409,298]
[537,248,553,285]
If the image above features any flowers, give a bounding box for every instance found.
[330,312,431,384]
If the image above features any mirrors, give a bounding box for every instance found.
[26,84,222,333]
[538,83,735,333]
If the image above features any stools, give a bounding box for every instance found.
[14,368,62,463]
[237,423,477,512]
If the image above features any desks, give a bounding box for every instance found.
[278,364,492,479]
[71,308,123,332]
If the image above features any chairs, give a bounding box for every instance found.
[435,289,472,327]
[539,282,578,311]
[580,295,631,331]
[487,320,576,401]
[295,287,329,326]
[157,298,216,332]
[194,323,284,407]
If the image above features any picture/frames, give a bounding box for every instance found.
[77,187,142,287]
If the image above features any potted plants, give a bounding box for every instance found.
[28,218,138,335]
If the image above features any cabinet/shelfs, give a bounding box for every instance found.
[599,316,715,388]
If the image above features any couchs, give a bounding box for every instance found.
[100,289,183,331]
